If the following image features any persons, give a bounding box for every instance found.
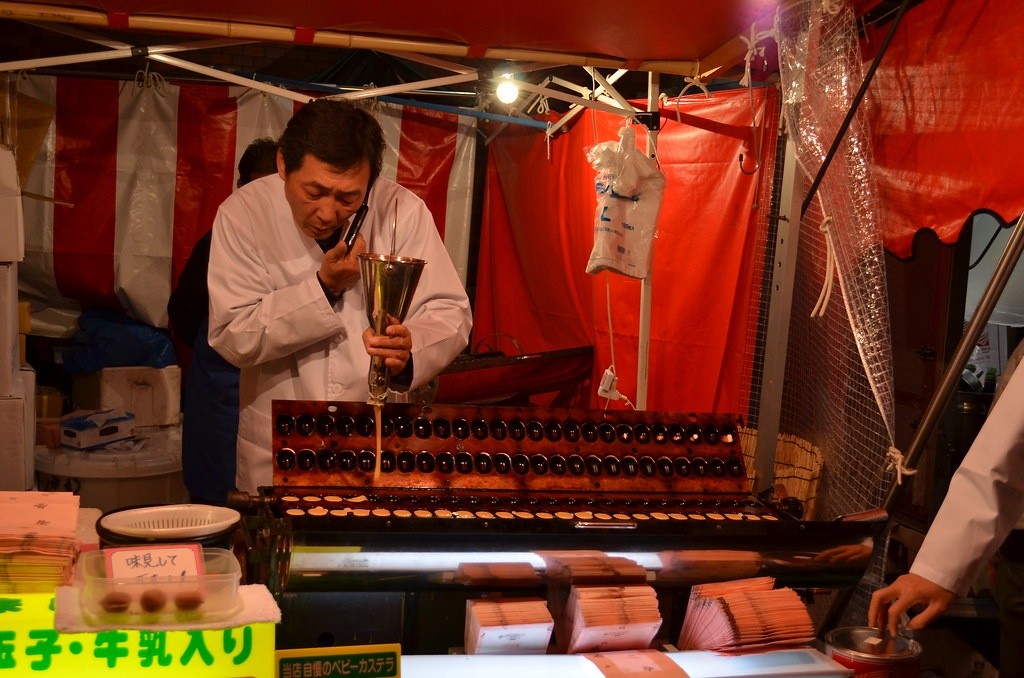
[168,138,279,508]
[867,357,1024,637]
[207,97,473,496]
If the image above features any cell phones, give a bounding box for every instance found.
[342,175,374,246]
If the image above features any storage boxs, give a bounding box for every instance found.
[0,143,62,491]
[85,364,181,429]
[74,547,243,623]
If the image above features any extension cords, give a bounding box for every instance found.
[597,386,620,400]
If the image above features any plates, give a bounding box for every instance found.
[100,504,240,539]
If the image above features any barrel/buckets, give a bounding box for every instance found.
[824,625,922,678]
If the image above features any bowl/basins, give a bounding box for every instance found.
[94,505,241,546]
[78,549,241,625]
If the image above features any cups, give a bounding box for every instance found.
[357,200,426,405]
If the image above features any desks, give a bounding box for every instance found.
[33,430,182,501]
